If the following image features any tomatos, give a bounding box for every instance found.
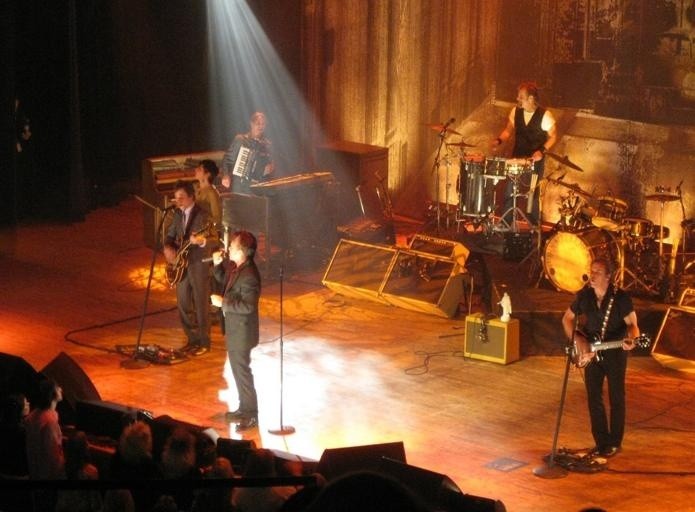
[141,151,229,253]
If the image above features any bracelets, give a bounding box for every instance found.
[496,137,501,145]
[538,146,546,156]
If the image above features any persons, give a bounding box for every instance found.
[3,391,32,478]
[105,415,276,481]
[214,112,274,193]
[563,256,640,456]
[309,472,431,511]
[211,232,262,433]
[57,429,100,482]
[162,181,222,355]
[14,99,33,157]
[192,159,226,324]
[28,382,66,480]
[488,82,558,225]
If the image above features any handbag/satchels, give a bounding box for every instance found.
[538,146,546,156]
[496,137,501,145]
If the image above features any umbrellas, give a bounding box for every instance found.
[603,447,618,457]
[593,448,601,455]
[193,346,208,355]
[183,344,199,351]
[240,417,257,428]
[226,411,242,419]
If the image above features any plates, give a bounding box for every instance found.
[316,140,388,217]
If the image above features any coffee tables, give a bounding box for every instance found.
[548,177,596,198]
[644,193,678,201]
[547,153,585,173]
[423,123,460,136]
[443,142,476,148]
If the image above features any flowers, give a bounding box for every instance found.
[163,204,176,211]
[201,253,229,264]
[577,282,591,298]
[442,118,455,132]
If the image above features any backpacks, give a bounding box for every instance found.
[462,175,506,219]
[486,157,506,173]
[622,218,654,240]
[504,160,535,176]
[543,227,620,292]
[591,199,627,231]
[459,156,486,176]
